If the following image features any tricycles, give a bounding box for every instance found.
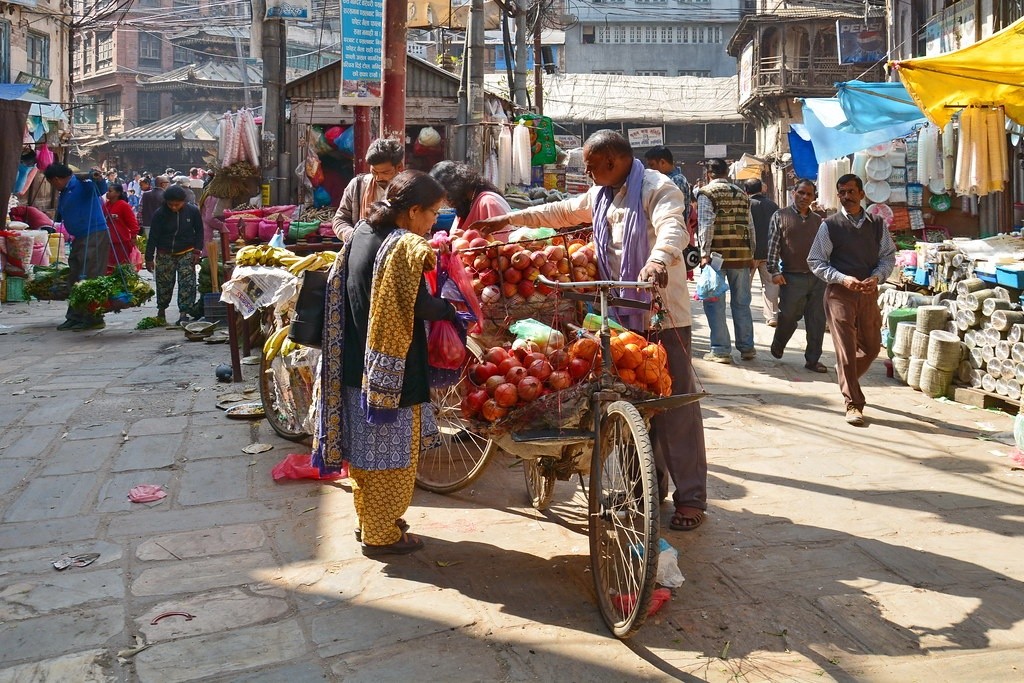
[412,273,661,641]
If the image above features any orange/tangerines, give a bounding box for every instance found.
[566,328,671,397]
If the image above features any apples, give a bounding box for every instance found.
[454,229,597,303]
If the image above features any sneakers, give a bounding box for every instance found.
[846,403,864,425]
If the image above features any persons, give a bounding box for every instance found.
[645,145,781,362]
[466,129,707,530]
[807,175,896,423]
[311,138,512,555]
[767,179,827,373]
[101,166,213,326]
[43,162,108,332]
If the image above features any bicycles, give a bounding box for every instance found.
[259,311,497,493]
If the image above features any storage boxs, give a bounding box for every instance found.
[893,226,1024,311]
[0,277,28,301]
[204,292,229,327]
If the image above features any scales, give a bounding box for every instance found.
[508,280,599,442]
[630,281,713,414]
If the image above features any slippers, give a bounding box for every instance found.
[771,329,783,359]
[805,361,826,373]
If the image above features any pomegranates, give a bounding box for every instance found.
[461,339,591,421]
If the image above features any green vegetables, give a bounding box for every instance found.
[71,264,152,318]
[22,261,69,302]
[135,317,167,329]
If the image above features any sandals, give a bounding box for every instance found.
[361,533,422,556]
[669,504,704,530]
[354,518,406,539]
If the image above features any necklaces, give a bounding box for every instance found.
[469,187,477,211]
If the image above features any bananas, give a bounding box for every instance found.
[263,325,297,361]
[235,244,336,276]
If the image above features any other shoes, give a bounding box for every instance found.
[71,321,106,332]
[175,315,189,325]
[741,347,757,359]
[57,319,85,331]
[702,352,730,362]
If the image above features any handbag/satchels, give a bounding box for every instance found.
[36,144,51,170]
[21,144,37,166]
[287,269,329,350]
[696,263,728,300]
[427,319,465,371]
